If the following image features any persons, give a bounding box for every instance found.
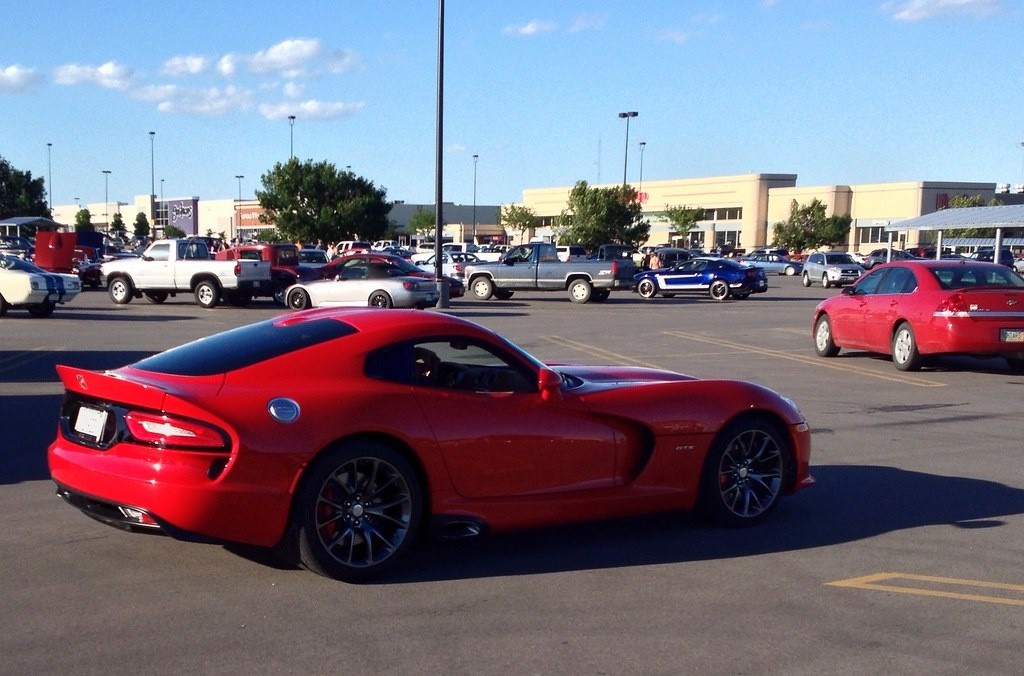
[315,239,338,262]
[210,239,230,254]
[643,247,662,270]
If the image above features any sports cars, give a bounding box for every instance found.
[46,305,817,583]
[283,262,441,312]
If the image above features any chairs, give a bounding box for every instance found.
[195,246,206,256]
[895,271,916,293]
[180,245,191,257]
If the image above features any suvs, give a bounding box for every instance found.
[737,245,790,264]
[801,250,866,289]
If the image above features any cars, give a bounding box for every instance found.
[0,231,516,305]
[0,252,82,319]
[847,242,1024,278]
[738,253,804,276]
[631,257,768,301]
[811,259,1024,371]
[547,240,747,292]
[776,251,809,266]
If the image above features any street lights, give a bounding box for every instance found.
[148,131,157,242]
[471,154,479,237]
[618,111,639,243]
[160,179,165,230]
[235,174,245,243]
[46,142,53,217]
[102,170,112,255]
[638,142,647,213]
[287,114,297,245]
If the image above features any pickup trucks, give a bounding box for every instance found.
[99,237,272,310]
[463,241,618,306]
